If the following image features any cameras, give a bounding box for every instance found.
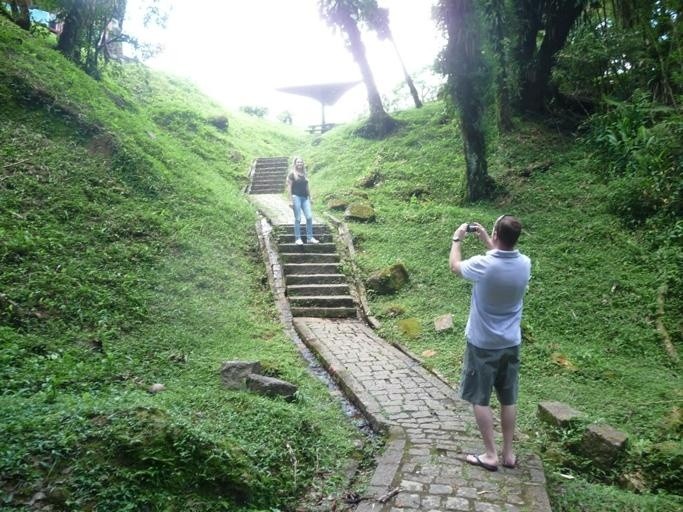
[467,225,478,232]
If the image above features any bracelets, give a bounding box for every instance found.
[450,237,461,242]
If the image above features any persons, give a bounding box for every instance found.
[287,156,319,245]
[447,215,533,471]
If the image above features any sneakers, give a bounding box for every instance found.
[295,240,304,246]
[307,238,319,244]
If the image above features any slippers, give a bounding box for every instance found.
[465,452,498,472]
[501,453,521,468]
